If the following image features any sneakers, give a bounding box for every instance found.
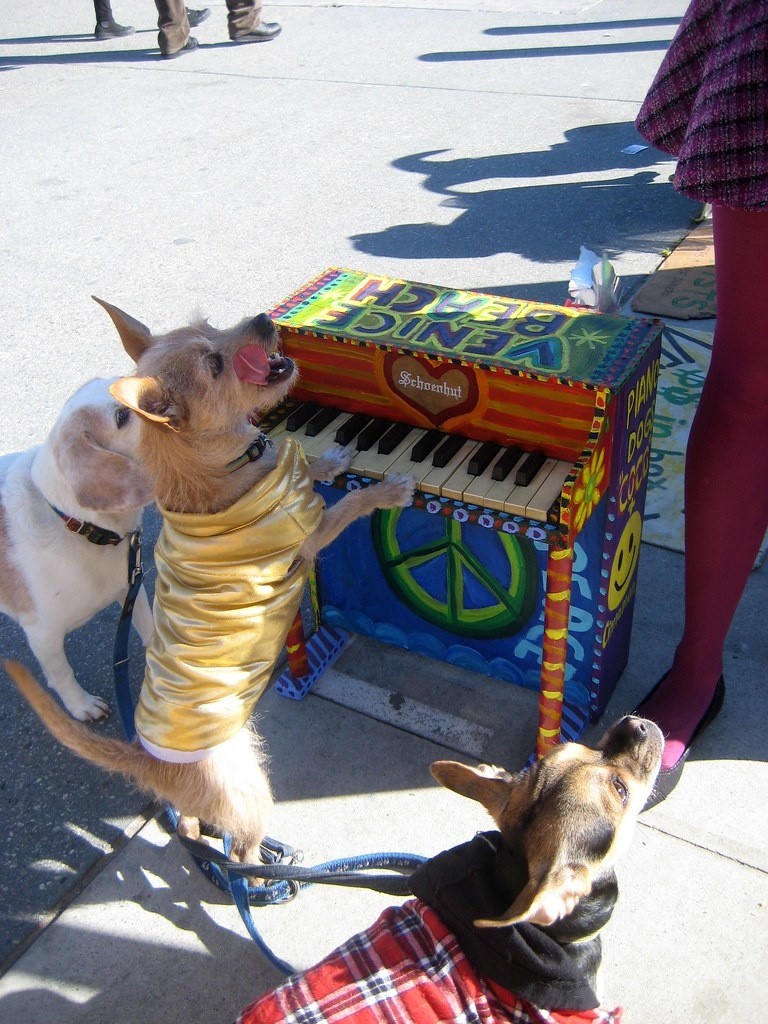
[93,19,135,41]
[185,6,211,27]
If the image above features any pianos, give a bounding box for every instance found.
[240,269,664,777]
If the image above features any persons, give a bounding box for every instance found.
[94,1,283,58]
[636,1,768,810]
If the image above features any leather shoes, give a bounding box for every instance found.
[230,21,281,42]
[622,662,726,816]
[163,37,199,59]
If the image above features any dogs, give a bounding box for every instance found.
[231,710,664,1024]
[1,296,417,898]
[1,376,153,729]
